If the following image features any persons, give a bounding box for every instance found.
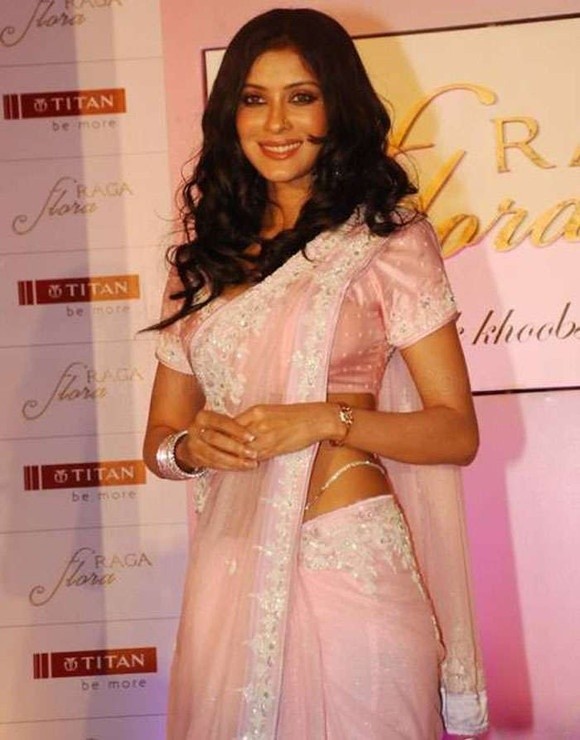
[139,8,490,740]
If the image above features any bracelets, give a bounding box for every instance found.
[154,429,209,480]
[329,400,353,446]
